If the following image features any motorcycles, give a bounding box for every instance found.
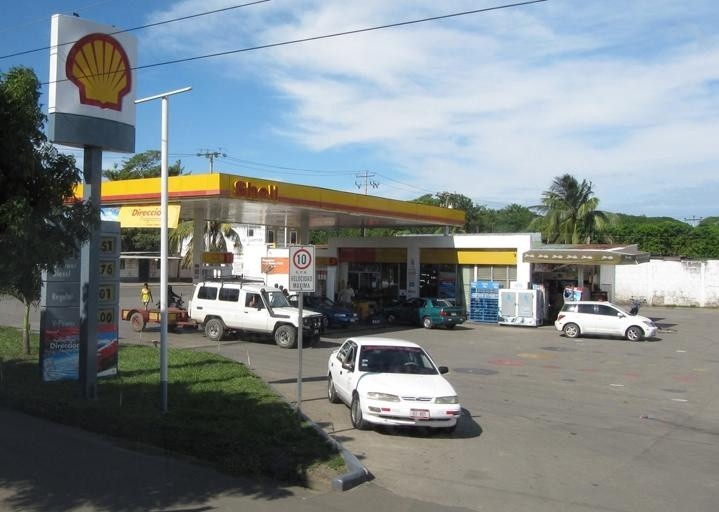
[156,294,186,311]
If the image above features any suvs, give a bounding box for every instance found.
[187,280,328,349]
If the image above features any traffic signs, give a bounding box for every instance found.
[289,245,315,292]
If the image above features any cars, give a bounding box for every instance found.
[286,294,359,328]
[326,336,462,434]
[554,299,658,341]
[381,296,469,330]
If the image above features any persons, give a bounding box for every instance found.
[337,281,356,310]
[167,285,180,308]
[139,283,154,311]
[249,294,258,307]
[595,294,604,303]
